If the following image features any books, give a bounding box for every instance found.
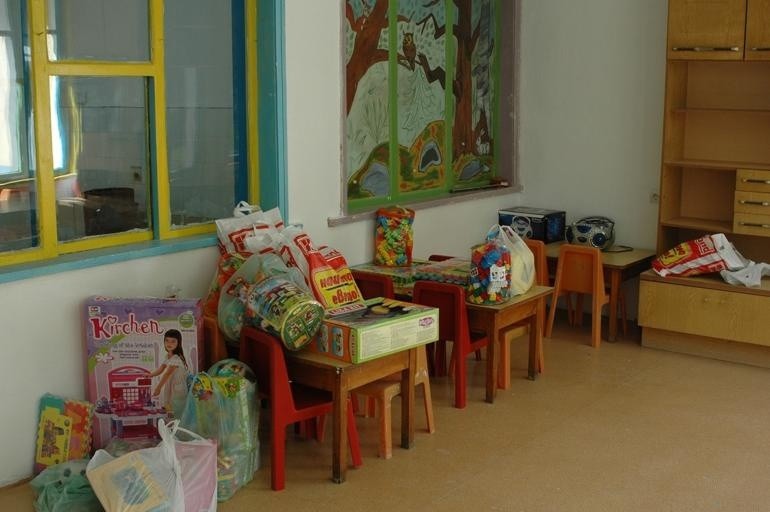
[86,437,218,511]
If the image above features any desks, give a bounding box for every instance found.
[284,347,417,486]
[544,239,657,342]
[348,258,557,406]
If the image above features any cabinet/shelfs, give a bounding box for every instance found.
[640,1,769,369]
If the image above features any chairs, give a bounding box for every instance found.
[352,270,394,300]
[548,244,610,349]
[349,344,435,461]
[497,240,546,391]
[200,315,228,368]
[412,279,487,410]
[239,325,363,491]
[524,239,548,287]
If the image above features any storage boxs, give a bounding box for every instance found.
[304,297,439,365]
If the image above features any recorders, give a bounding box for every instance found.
[565,217,616,250]
[509,215,535,239]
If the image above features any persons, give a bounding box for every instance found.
[146,329,192,419]
